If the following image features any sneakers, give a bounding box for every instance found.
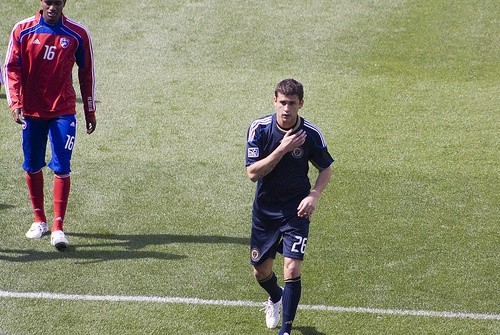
[260,286,284,329]
[26,222,49,239]
[51,230,68,249]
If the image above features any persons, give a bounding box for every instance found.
[246,79,334,335]
[3,0,98,247]
